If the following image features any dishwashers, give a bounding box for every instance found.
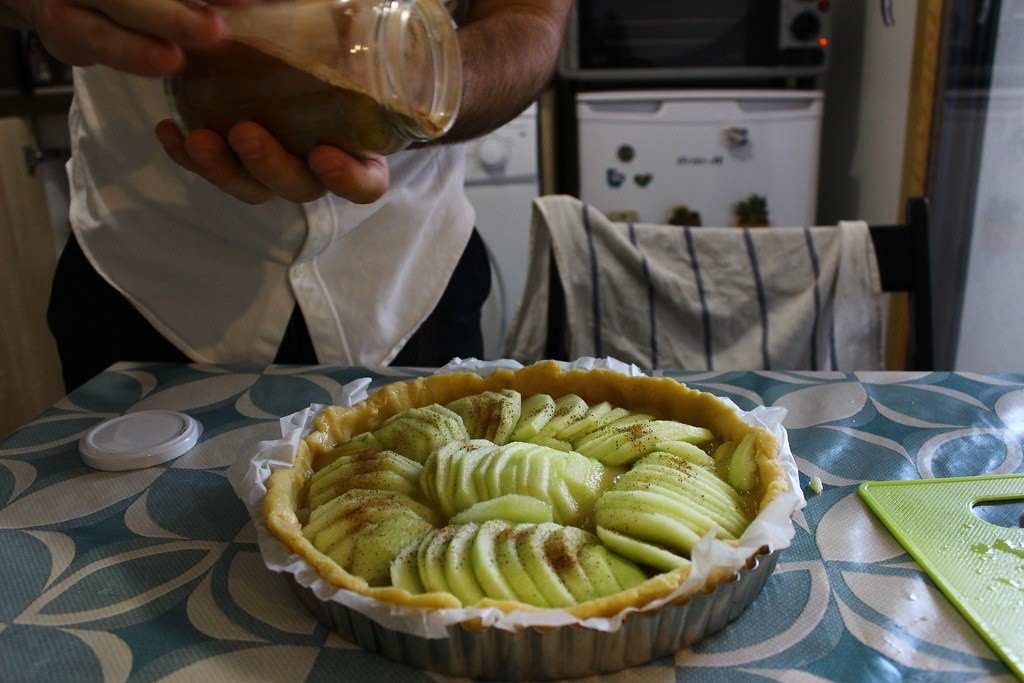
[575,93,829,224]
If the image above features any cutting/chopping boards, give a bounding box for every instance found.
[859,472,1024,683]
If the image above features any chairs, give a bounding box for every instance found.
[539,196,935,372]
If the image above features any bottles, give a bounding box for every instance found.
[161,0,461,160]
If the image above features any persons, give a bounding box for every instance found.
[0,0,572,396]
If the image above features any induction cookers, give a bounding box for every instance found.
[556,0,848,92]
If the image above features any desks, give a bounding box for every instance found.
[0,361,1024,683]
